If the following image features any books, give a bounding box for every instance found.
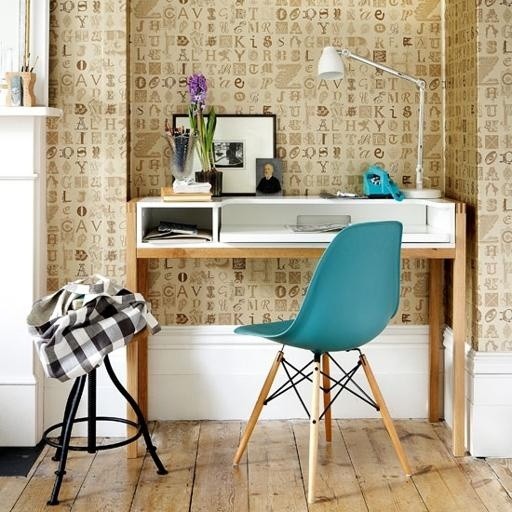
[156,221,199,235]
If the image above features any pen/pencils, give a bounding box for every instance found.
[165,118,196,172]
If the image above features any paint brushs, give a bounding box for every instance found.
[22,53,39,72]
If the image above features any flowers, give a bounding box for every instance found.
[186,73,216,172]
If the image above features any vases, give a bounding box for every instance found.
[194,170,223,198]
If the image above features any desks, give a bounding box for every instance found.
[125,196,467,458]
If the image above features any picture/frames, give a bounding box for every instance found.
[172,113,277,197]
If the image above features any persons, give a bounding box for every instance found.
[256,161,281,195]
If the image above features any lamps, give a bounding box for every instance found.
[317,45,443,199]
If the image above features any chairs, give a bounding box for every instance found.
[234,221,412,504]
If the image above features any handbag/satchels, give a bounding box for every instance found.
[26,274,161,383]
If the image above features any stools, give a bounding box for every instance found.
[35,315,168,506]
